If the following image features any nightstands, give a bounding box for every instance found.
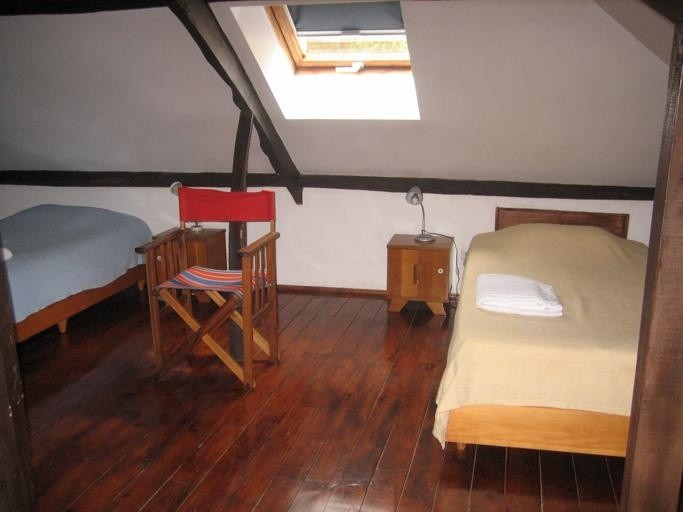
[152,226,227,303]
[386,234,455,317]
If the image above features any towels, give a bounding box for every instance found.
[2,247,13,262]
[476,273,564,317]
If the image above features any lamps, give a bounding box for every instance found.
[170,181,204,232]
[405,186,436,243]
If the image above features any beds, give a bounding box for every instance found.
[432,206,648,458]
[0,203,155,345]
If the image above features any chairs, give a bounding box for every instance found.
[134,187,281,392]
